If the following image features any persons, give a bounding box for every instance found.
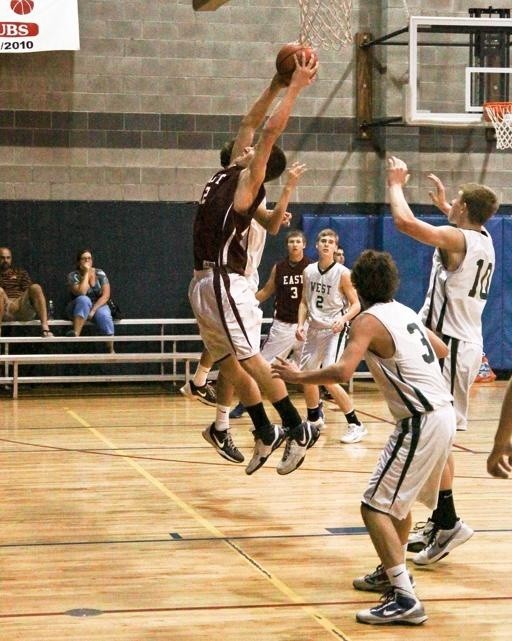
[271,248,459,626]
[0,246,54,337]
[386,158,512,563]
[177,52,368,473]
[66,248,116,354]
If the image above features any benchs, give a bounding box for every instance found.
[1,314,376,404]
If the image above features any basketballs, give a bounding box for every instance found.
[276,42,317,84]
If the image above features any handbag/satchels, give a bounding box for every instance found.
[107,298,121,320]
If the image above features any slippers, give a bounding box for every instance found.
[40,329,54,338]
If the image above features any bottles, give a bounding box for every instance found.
[47,301,55,321]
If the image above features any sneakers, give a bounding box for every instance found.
[336,420,370,444]
[351,565,429,627]
[178,379,325,474]
[396,518,476,566]
